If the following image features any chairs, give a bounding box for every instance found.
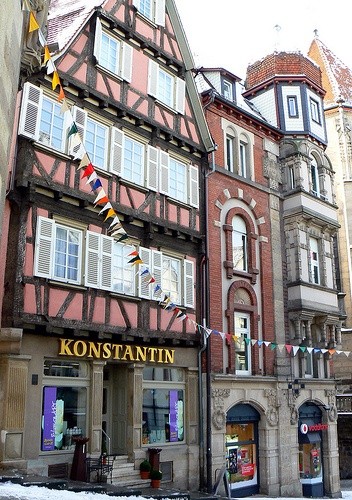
[85,456,114,484]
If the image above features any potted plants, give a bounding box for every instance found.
[140,460,152,480]
[150,469,163,488]
